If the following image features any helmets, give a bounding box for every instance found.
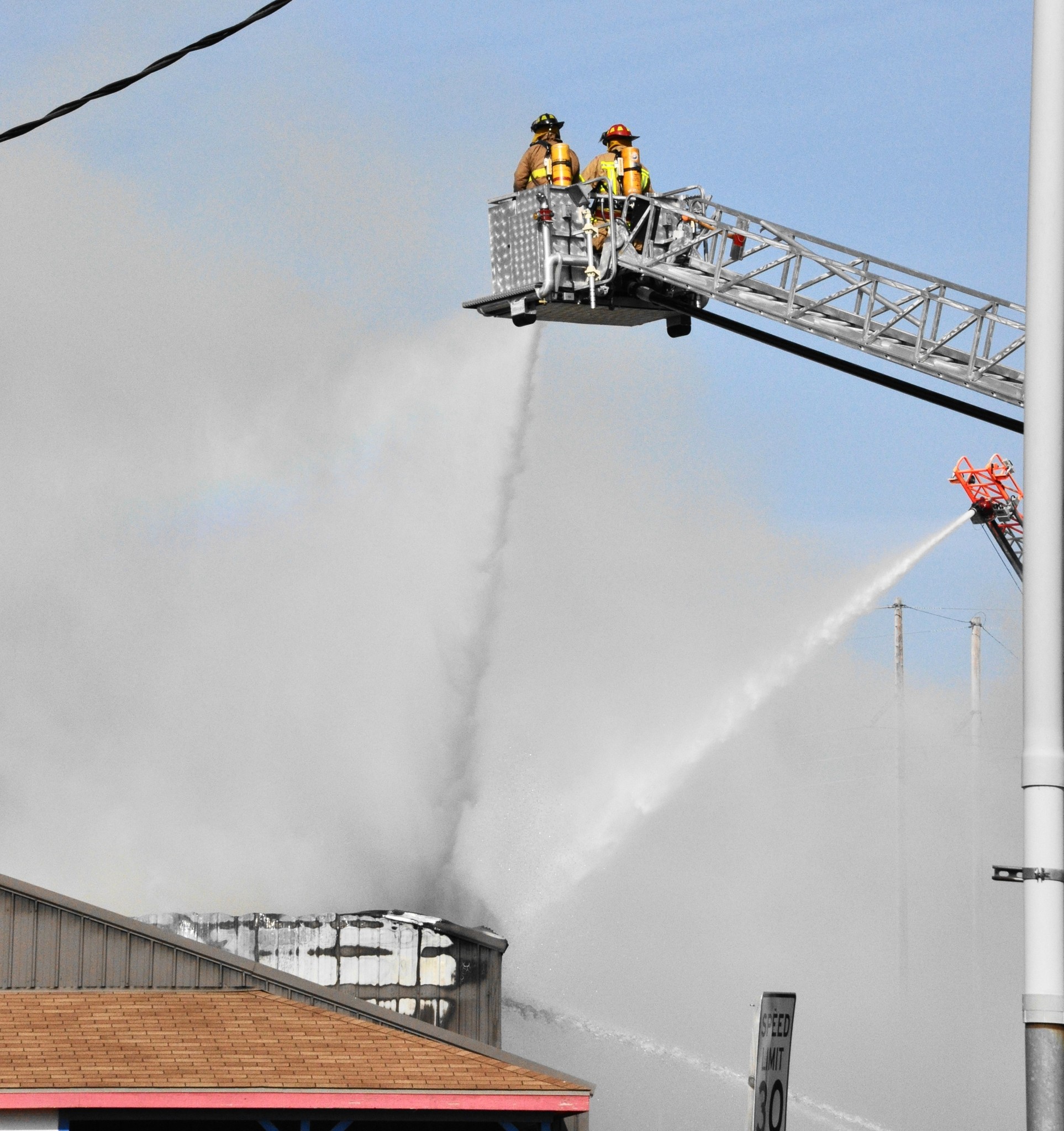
[531,114,565,133]
[602,124,640,146]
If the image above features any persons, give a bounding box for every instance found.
[514,113,656,258]
